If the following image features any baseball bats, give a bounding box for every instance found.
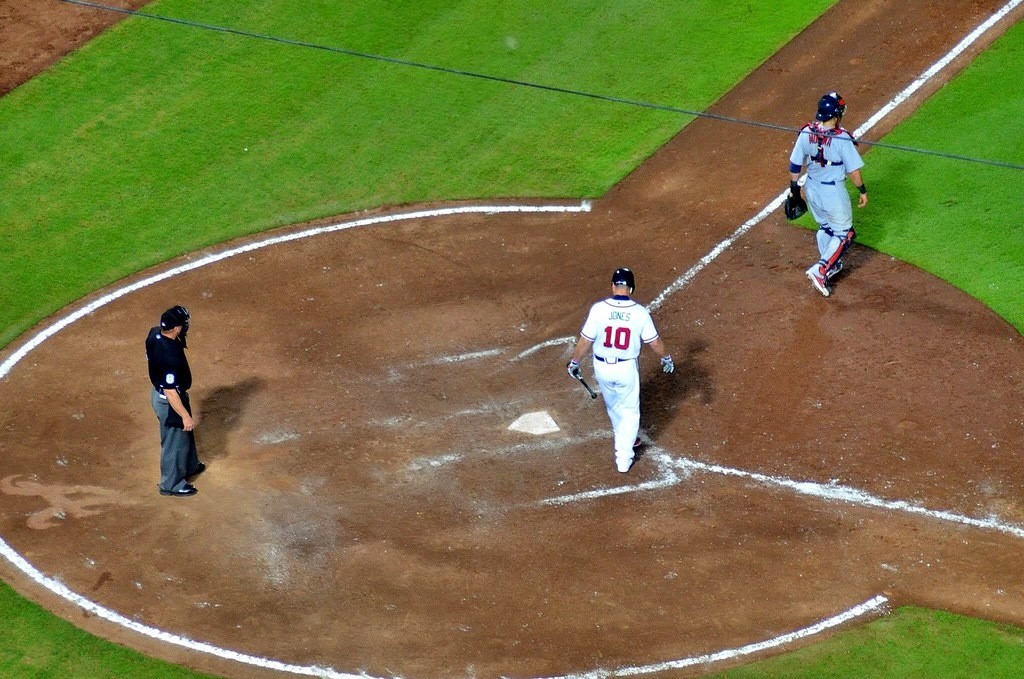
[566,361,597,399]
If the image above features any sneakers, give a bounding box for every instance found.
[826,258,844,280]
[805,264,829,298]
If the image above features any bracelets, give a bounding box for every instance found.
[858,185,867,194]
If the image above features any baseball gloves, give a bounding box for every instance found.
[783,193,809,220]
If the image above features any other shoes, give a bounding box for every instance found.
[618,458,635,473]
[632,435,642,448]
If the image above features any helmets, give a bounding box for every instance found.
[611,267,635,294]
[815,91,846,122]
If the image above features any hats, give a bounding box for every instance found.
[160,306,190,331]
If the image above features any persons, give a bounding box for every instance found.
[786,92,867,298]
[568,269,674,471]
[146,305,204,497]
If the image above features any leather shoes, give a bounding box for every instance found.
[159,482,198,496]
[192,461,205,475]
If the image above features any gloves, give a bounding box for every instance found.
[567,359,581,380]
[660,354,674,373]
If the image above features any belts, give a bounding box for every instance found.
[594,354,632,362]
[155,387,186,396]
[808,175,835,185]
